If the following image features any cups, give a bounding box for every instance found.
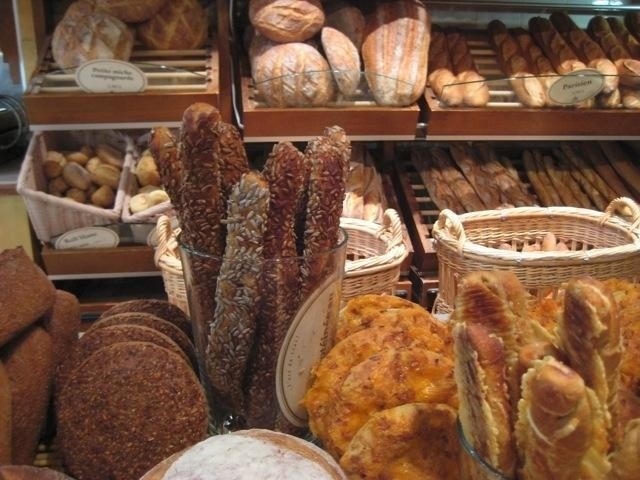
[453,412,508,480]
[174,225,350,445]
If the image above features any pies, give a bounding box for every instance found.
[310,295,461,475]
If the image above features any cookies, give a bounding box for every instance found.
[50,298,214,474]
[51,1,214,77]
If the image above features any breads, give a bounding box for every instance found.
[451,267,640,475]
[406,138,640,221]
[14,129,135,243]
[429,13,638,109]
[122,129,177,243]
[248,1,431,107]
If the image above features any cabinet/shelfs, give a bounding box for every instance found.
[1,0,640,329]
